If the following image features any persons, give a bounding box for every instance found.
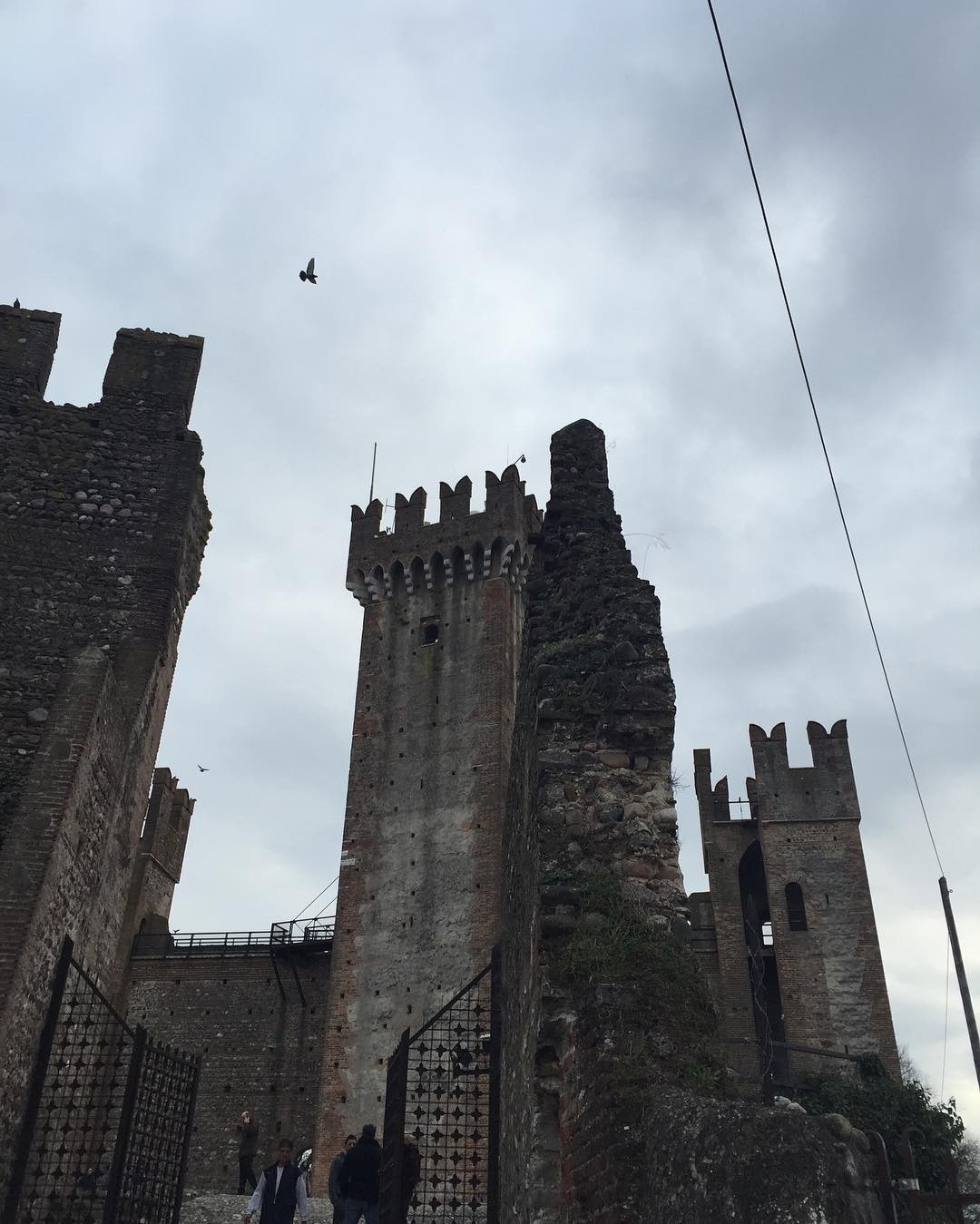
[237,1111,258,1195]
[328,1124,421,1224]
[243,1139,309,1224]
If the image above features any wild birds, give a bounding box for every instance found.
[299,258,318,284]
[172,929,180,933]
[774,1096,792,1108]
[197,764,210,772]
[13,298,20,309]
